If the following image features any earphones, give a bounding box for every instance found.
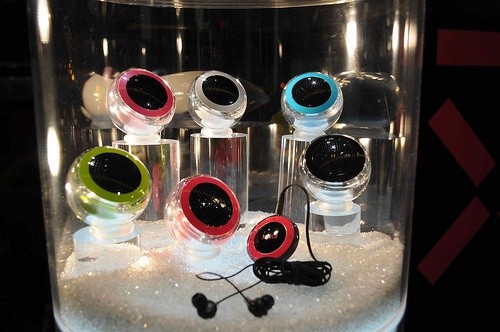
[248,293,274,320]
[191,292,218,320]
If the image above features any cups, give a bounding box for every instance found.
[26,1,424,332]
[112,138,179,247]
[189,132,250,214]
[277,134,320,225]
